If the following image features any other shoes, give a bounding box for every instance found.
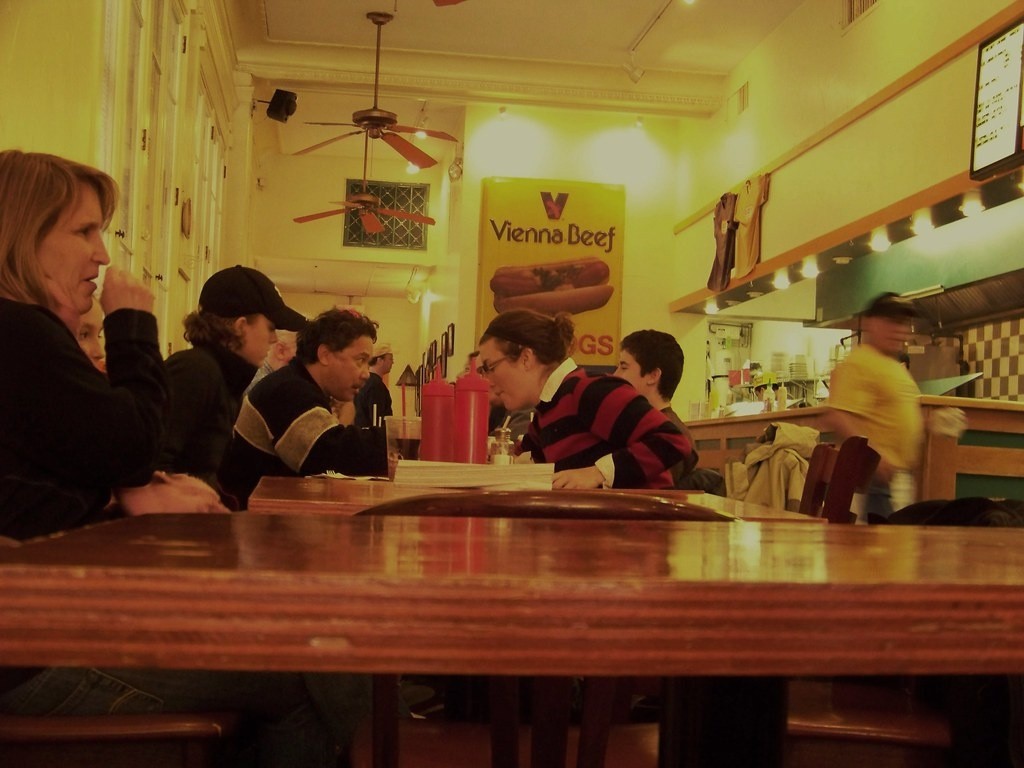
[408,694,476,721]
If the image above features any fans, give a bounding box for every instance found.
[290,130,436,236]
[289,14,460,170]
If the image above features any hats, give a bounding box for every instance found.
[199,264,306,332]
[754,372,777,388]
[864,291,920,319]
[371,342,393,357]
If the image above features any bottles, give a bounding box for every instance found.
[764,378,775,410]
[420,361,455,462]
[778,382,787,411]
[719,405,725,418]
[452,355,490,464]
[487,426,513,465]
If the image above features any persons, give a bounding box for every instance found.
[244,330,296,393]
[354,344,395,427]
[825,292,922,525]
[479,310,691,490]
[234,308,387,500]
[754,372,780,401]
[162,266,310,505]
[78,296,105,366]
[0,152,337,768]
[458,352,537,441]
[614,329,699,481]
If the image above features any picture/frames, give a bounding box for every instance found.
[414,322,456,417]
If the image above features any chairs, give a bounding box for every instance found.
[0,699,241,768]
[776,680,956,768]
[797,436,882,527]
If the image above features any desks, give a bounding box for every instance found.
[1,469,1023,768]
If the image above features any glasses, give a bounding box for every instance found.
[476,350,521,381]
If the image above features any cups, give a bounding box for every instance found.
[385,417,421,483]
